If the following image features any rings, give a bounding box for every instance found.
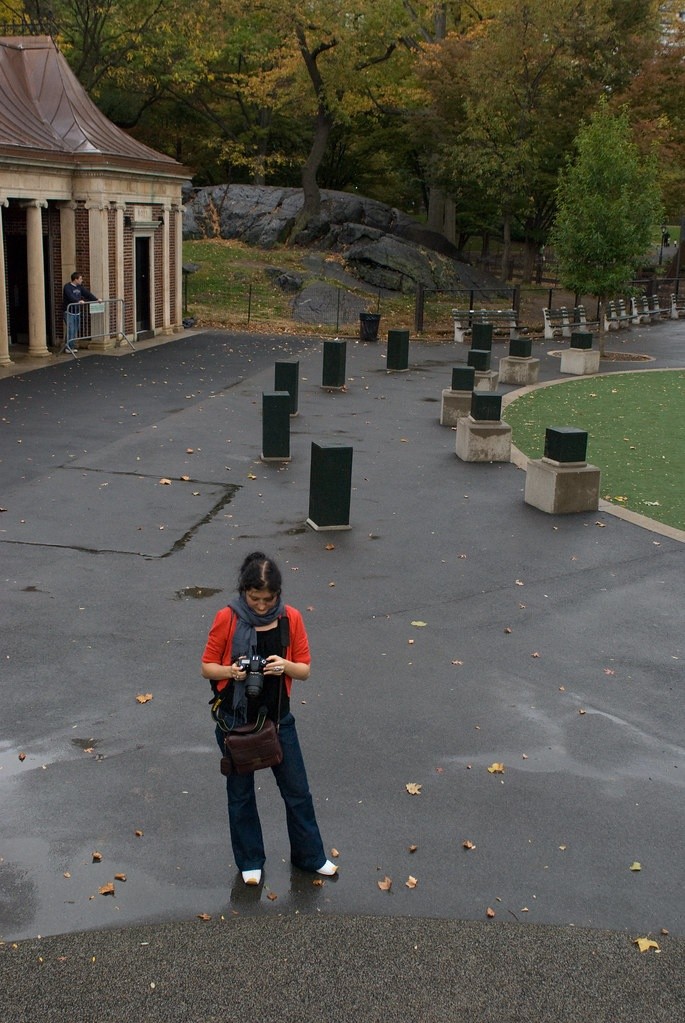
[274,667,280,671]
[237,675,239,678]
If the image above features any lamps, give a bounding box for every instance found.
[124,216,131,227]
[158,216,164,226]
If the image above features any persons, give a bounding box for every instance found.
[200,551,339,884]
[663,232,677,247]
[62,272,102,353]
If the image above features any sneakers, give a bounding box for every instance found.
[241,869,262,885]
[315,860,339,875]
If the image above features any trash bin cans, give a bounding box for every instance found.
[359,312,381,341]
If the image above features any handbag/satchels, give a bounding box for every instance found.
[220,719,283,777]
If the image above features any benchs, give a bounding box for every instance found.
[451,307,530,342]
[631,294,670,325]
[670,292,685,320]
[599,299,639,333]
[542,304,600,339]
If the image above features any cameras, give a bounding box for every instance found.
[238,655,273,697]
